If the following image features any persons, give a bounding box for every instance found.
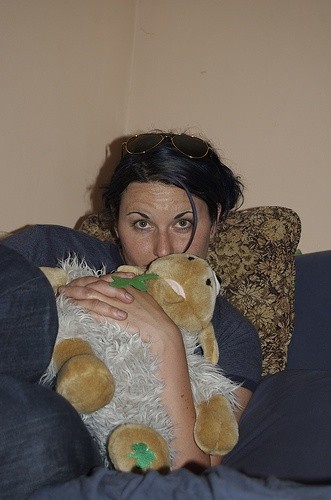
[0,133,262,474]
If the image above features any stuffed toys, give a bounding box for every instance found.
[33,253,244,472]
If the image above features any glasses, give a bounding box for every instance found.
[121,133,219,159]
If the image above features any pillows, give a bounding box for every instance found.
[73,206,302,382]
